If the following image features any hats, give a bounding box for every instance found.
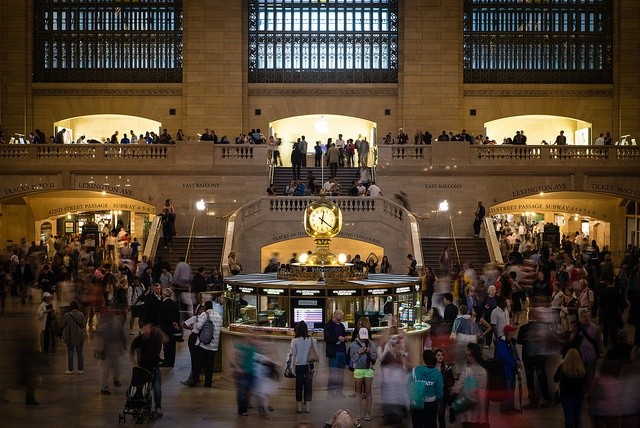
[42,291,52,298]
[359,328,368,339]
[504,325,518,333]
[452,367,479,411]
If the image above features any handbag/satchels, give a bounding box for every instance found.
[307,334,320,377]
[284,352,297,378]
[407,367,426,410]
[198,311,214,345]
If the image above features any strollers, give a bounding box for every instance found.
[119,367,157,424]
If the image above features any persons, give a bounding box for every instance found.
[160,198,178,236]
[604,132,612,145]
[112,278,126,328]
[560,287,577,331]
[294,178,305,211]
[144,255,222,301]
[9,248,21,297]
[327,143,341,177]
[91,310,126,395]
[577,279,594,322]
[32,228,80,300]
[239,291,250,308]
[292,420,313,428]
[141,282,163,323]
[423,131,432,145]
[365,181,384,196]
[314,141,322,167]
[38,293,59,355]
[473,201,485,238]
[285,181,298,196]
[490,297,511,360]
[496,285,551,309]
[324,409,364,428]
[291,146,302,178]
[126,275,146,335]
[353,254,369,274]
[494,325,524,415]
[553,348,588,428]
[406,254,417,274]
[156,287,181,369]
[29,127,185,157]
[182,306,208,382]
[348,180,359,211]
[325,177,342,203]
[491,215,640,285]
[324,309,349,399]
[344,140,351,167]
[301,136,307,168]
[17,319,47,406]
[553,130,566,145]
[355,134,370,168]
[480,262,491,298]
[451,347,490,427]
[200,128,211,141]
[267,184,279,211]
[542,140,548,145]
[396,127,409,159]
[210,130,217,141]
[378,325,417,427]
[463,342,491,413]
[366,253,379,273]
[180,301,223,388]
[380,256,392,274]
[595,133,604,146]
[433,350,455,428]
[479,286,498,349]
[0,240,14,313]
[518,309,554,409]
[449,129,526,144]
[80,221,144,278]
[592,284,640,428]
[349,327,378,422]
[383,132,396,143]
[357,180,366,211]
[161,209,175,254]
[551,281,566,324]
[324,177,335,195]
[348,139,355,168]
[227,331,256,417]
[336,134,345,168]
[229,250,242,275]
[80,279,111,309]
[13,235,32,259]
[130,322,169,418]
[326,138,334,168]
[567,304,603,385]
[420,245,479,351]
[220,128,282,166]
[14,259,32,303]
[306,171,316,195]
[314,180,320,195]
[409,349,444,428]
[0,130,6,144]
[438,130,448,141]
[414,130,424,159]
[293,136,301,149]
[264,251,356,275]
[252,354,283,418]
[58,300,87,375]
[359,161,371,187]
[284,321,320,413]
[351,316,372,399]
[390,191,411,219]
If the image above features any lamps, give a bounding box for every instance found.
[102,191,106,196]
[336,254,348,264]
[298,253,310,264]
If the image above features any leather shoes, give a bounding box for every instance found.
[25,398,40,405]
[179,380,195,387]
[159,363,174,367]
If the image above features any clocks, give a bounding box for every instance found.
[299,189,347,264]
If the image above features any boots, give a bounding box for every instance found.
[304,400,312,413]
[296,401,303,413]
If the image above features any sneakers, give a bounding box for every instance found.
[101,388,111,395]
[77,369,84,373]
[64,368,73,374]
[364,413,371,421]
